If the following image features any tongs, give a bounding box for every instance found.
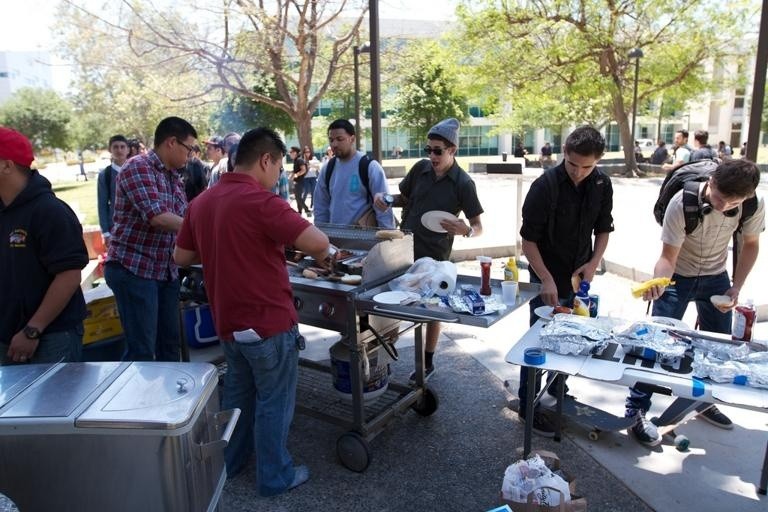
[328,246,339,277]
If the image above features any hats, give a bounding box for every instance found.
[202,136,224,145]
[0,128,34,167]
[426,117,459,147]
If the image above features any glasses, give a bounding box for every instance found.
[175,138,194,157]
[424,146,445,155]
[269,154,284,174]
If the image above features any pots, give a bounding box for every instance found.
[303,256,318,267]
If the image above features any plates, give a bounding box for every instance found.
[421,211,457,233]
[373,288,420,304]
[534,306,574,321]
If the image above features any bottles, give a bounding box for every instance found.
[572,280,590,316]
[630,276,677,299]
[502,257,520,299]
[475,255,493,297]
[590,293,598,319]
[732,304,760,345]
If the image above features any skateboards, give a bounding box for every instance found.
[650,397,715,452]
[506,379,639,440]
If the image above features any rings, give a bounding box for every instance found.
[20,356,27,361]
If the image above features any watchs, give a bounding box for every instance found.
[463,227,475,240]
[21,324,43,342]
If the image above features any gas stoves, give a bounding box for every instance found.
[178,228,387,333]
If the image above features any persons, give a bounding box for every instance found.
[287,147,310,218]
[373,118,484,387]
[514,140,528,160]
[718,139,733,159]
[301,146,319,210]
[311,118,399,231]
[689,130,718,161]
[183,132,288,200]
[662,129,690,170]
[320,146,334,169]
[128,136,148,158]
[539,142,552,173]
[634,140,644,160]
[98,134,131,248]
[740,141,748,155]
[518,127,615,439]
[0,126,89,365]
[171,128,337,495]
[648,141,669,163]
[625,159,766,447]
[105,116,198,362]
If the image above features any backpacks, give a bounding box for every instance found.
[652,157,760,236]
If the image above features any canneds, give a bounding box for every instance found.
[383,195,394,213]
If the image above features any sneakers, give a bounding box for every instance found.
[698,402,735,429]
[289,463,310,490]
[628,409,661,449]
[410,364,436,385]
[519,407,557,438]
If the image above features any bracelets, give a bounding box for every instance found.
[293,173,298,179]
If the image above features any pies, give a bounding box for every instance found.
[340,274,362,284]
[302,269,317,278]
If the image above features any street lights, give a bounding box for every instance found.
[354,41,371,150]
[627,47,643,140]
[625,43,645,175]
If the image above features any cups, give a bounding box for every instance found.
[501,280,518,306]
